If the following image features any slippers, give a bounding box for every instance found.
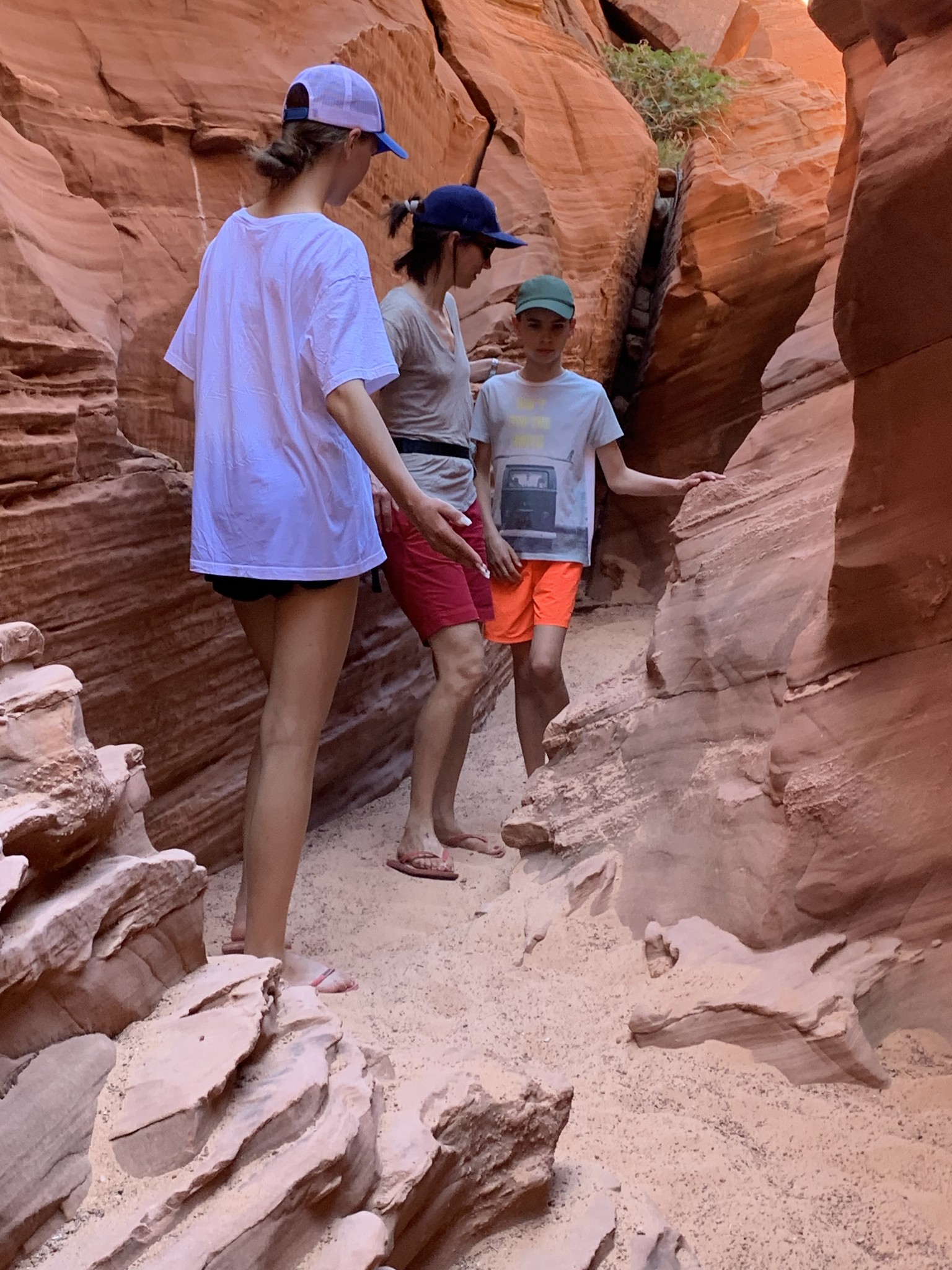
[223,938,292,957]
[386,845,460,881]
[440,833,505,859]
[279,956,358,993]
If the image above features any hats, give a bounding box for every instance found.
[414,184,528,249]
[283,63,408,160]
[514,275,575,320]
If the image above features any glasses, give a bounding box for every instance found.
[467,238,496,258]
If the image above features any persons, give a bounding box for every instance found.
[165,63,492,991]
[369,186,528,878]
[467,275,728,806]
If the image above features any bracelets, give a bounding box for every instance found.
[489,358,498,377]
